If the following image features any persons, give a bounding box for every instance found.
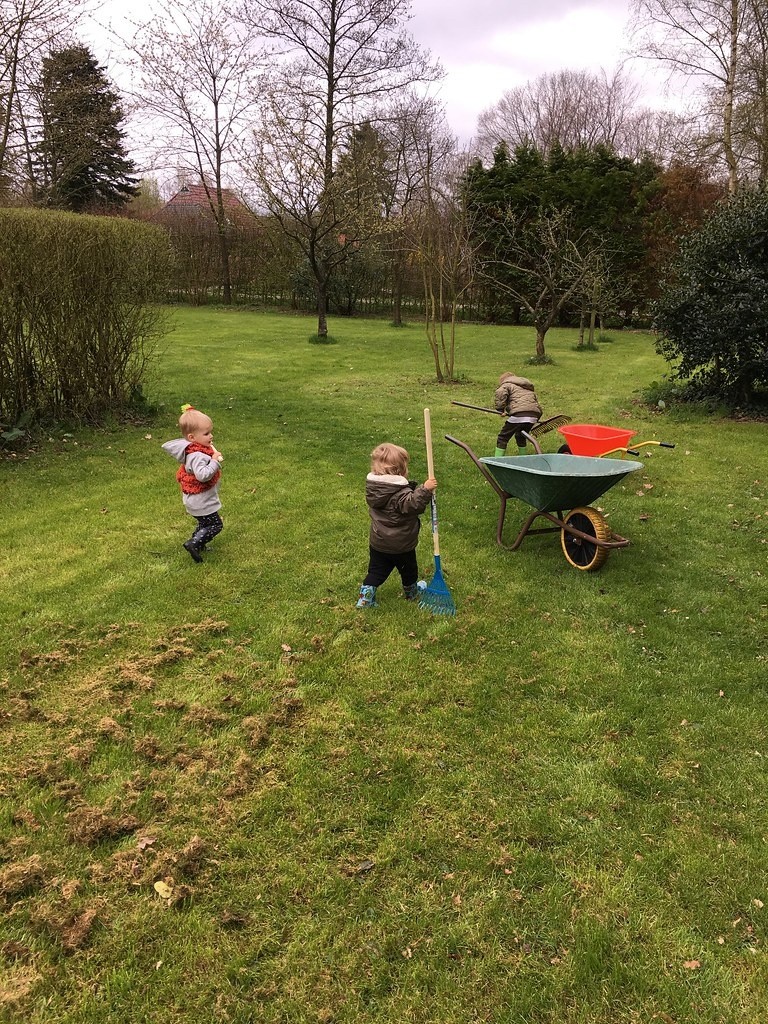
[493,372,545,456]
[162,406,223,564]
[356,442,437,610]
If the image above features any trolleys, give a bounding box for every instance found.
[442,430,647,569]
[557,420,676,463]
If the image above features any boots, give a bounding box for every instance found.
[192,532,213,553]
[404,580,428,602]
[495,447,505,457]
[183,527,212,562]
[518,447,526,455]
[356,585,380,610]
